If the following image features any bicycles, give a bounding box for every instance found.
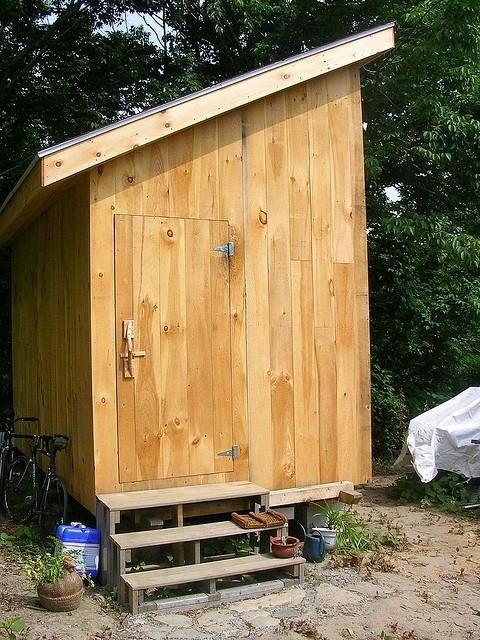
[0,412,71,535]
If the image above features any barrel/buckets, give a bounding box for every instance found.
[61,525,102,578]
[54,522,84,555]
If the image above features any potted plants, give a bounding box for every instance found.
[20,536,95,613]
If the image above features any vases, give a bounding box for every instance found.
[271,536,300,558]
